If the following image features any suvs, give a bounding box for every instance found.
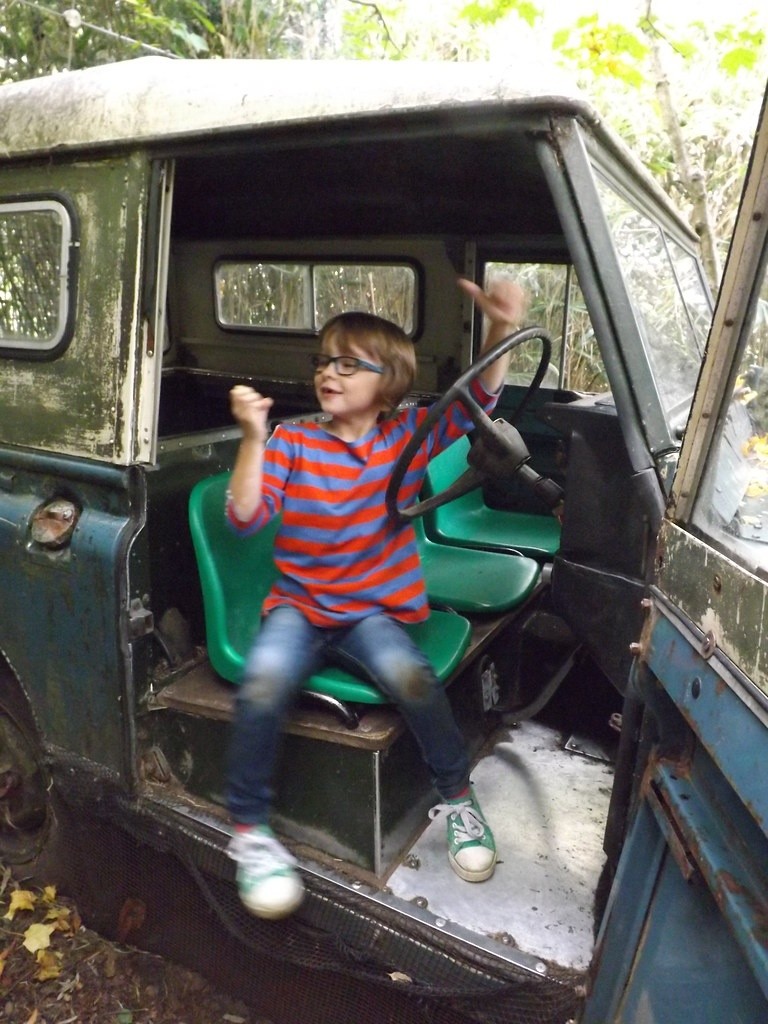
[0,55,768,1024]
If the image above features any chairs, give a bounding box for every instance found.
[425,434,567,553]
[412,485,538,614]
[186,474,472,706]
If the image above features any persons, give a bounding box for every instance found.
[224,278,522,919]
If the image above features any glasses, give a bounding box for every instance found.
[305,351,385,377]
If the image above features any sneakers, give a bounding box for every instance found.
[429,782,497,883]
[222,822,307,920]
